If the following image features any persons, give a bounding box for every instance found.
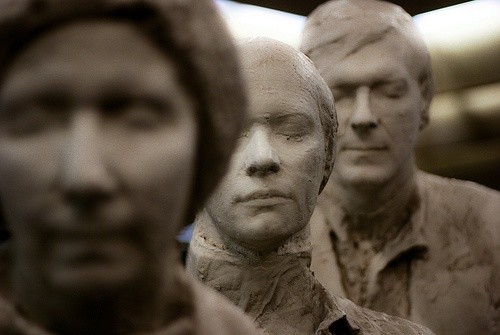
[180,33,436,334]
[1,1,270,335]
[292,1,500,334]
